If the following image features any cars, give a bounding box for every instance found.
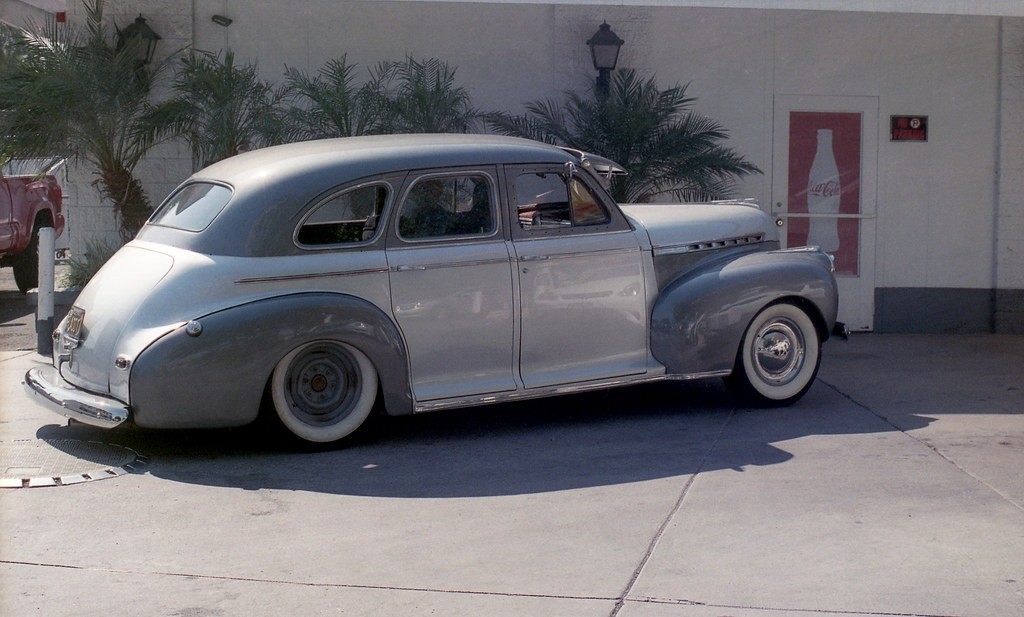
[20,133,849,454]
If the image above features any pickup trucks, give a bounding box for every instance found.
[0,161,66,294]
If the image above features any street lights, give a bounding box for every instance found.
[585,17,626,114]
